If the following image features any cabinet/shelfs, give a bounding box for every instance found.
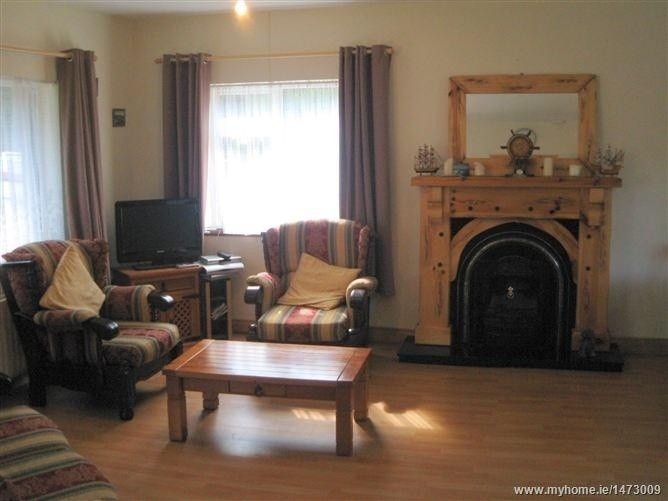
[112,273,235,341]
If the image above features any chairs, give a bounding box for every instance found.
[1,400,123,501]
[1,238,184,421]
[244,219,380,348]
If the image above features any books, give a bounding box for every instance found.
[200,254,241,265]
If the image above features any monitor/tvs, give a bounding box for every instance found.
[115,198,202,270]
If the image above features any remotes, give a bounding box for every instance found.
[217,251,231,259]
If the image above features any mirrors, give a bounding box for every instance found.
[447,73,601,176]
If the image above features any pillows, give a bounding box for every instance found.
[38,242,107,316]
[276,251,364,313]
